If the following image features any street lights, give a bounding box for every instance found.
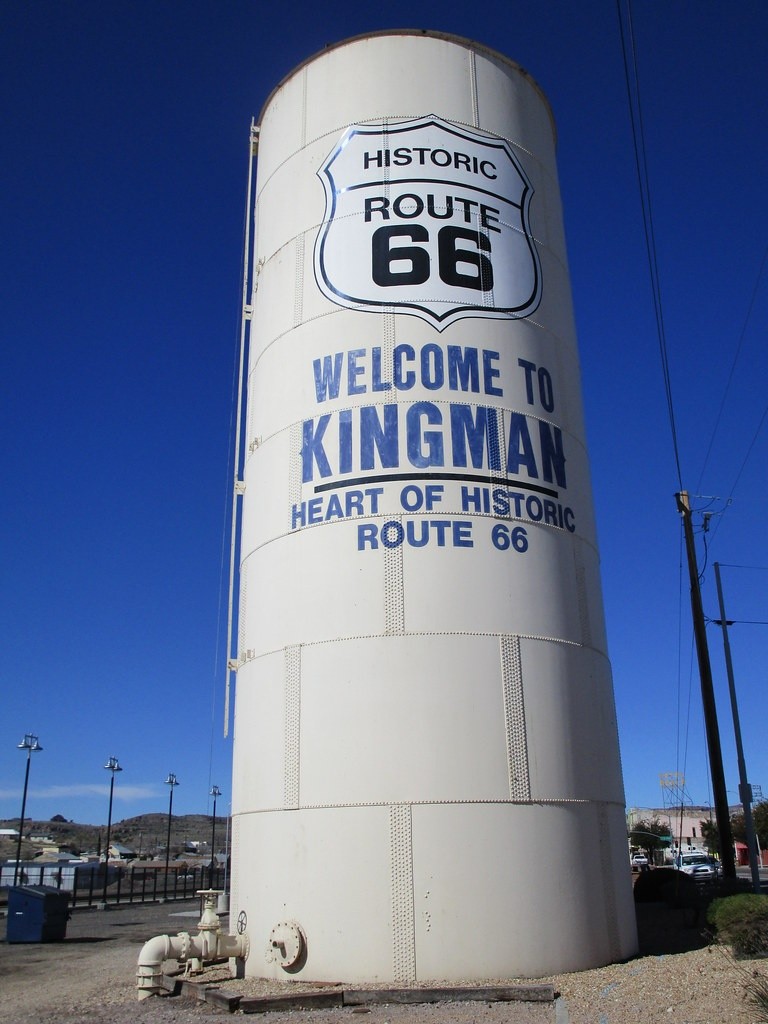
[97,755,124,911]
[209,785,222,890]
[11,732,44,887]
[161,772,183,901]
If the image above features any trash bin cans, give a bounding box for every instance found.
[5,885,69,944]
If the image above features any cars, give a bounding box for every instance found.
[631,854,649,867]
[707,855,722,873]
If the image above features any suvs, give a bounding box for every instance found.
[673,852,718,883]
